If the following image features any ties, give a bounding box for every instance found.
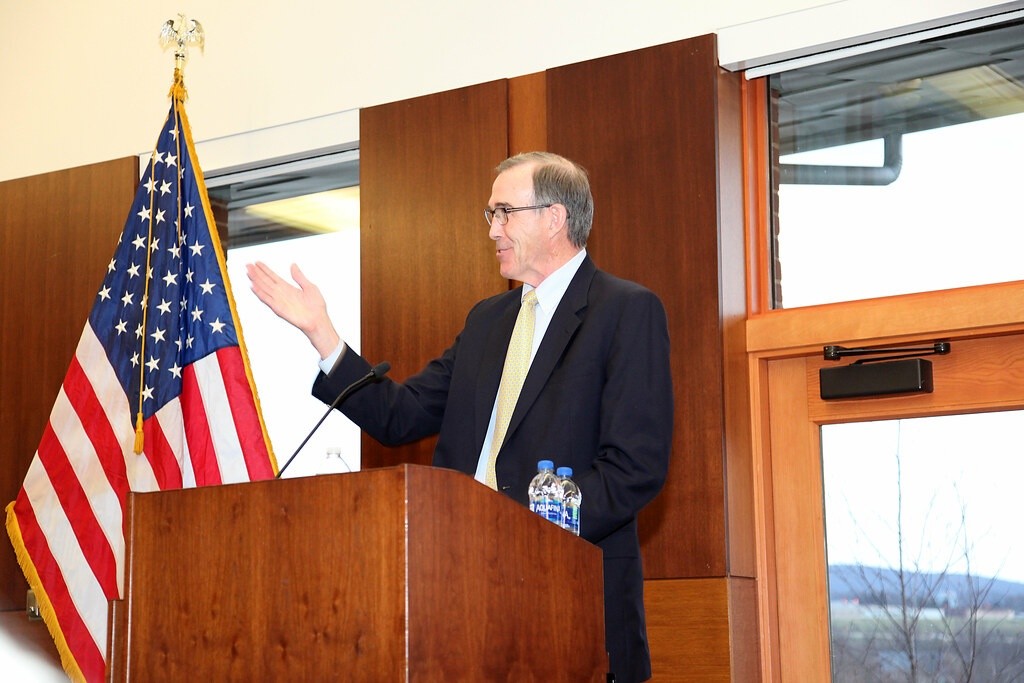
[485,290,539,491]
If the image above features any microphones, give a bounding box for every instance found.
[275,360,392,479]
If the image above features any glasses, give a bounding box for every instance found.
[483,204,570,226]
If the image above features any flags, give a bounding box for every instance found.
[5,72,280,683]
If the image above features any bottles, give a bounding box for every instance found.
[527,460,564,528]
[557,467,582,536]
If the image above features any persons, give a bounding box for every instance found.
[246,151,674,683]
